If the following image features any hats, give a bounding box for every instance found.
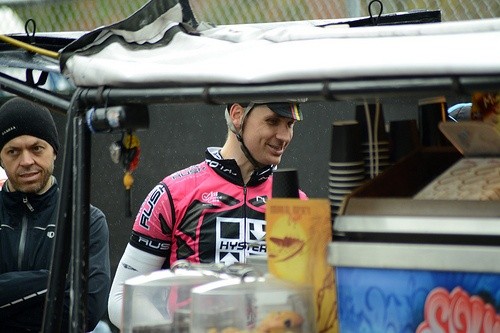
[227,101,304,121]
[0,97,60,169]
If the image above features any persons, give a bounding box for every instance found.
[448,91,500,136]
[107,100,311,333]
[0,94,112,333]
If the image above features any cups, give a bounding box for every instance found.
[328,96,449,226]
[272,169,299,198]
